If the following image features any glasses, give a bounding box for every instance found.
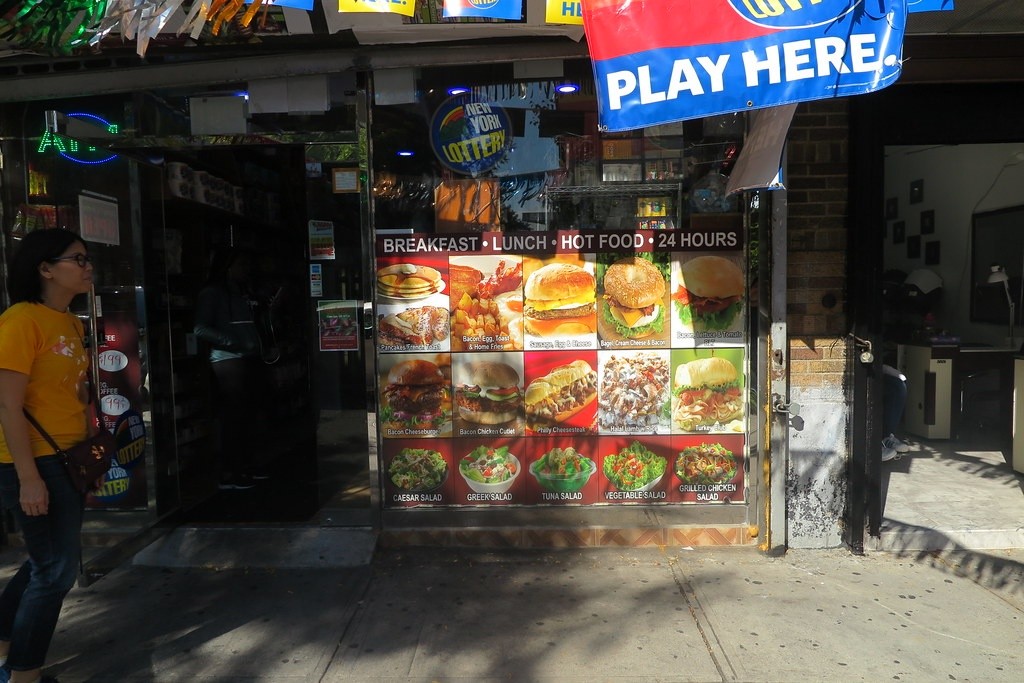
[45,253,93,268]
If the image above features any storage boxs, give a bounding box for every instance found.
[603,164,642,181]
[644,160,685,180]
[635,197,673,217]
[317,300,363,352]
[189,97,261,135]
[644,150,683,159]
[641,137,684,149]
[643,123,684,137]
[601,140,643,160]
[432,177,500,235]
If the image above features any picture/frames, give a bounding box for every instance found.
[910,179,922,205]
[906,235,920,258]
[892,221,905,243]
[886,197,898,219]
[921,209,935,234]
[331,168,362,194]
[925,242,939,264]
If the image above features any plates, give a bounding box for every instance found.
[376,291,450,352]
[449,255,524,352]
[377,279,446,304]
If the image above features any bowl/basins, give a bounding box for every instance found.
[673,451,737,494]
[458,448,521,496]
[389,450,449,493]
[529,457,597,492]
[603,467,666,495]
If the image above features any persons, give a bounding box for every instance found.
[846,347,909,461]
[193,248,271,489]
[0,228,103,682]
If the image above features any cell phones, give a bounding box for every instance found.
[272,287,285,305]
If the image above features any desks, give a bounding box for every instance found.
[958,346,1019,410]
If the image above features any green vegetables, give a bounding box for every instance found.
[386,441,738,493]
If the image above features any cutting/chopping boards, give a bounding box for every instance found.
[596,277,671,348]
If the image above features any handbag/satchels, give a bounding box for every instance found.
[60,428,115,481]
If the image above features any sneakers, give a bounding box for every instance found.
[882,432,909,462]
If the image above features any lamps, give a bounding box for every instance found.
[989,265,1017,349]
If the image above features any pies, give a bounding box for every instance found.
[375,263,441,299]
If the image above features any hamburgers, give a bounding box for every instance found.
[382,359,448,431]
[672,255,747,333]
[602,257,666,340]
[671,357,742,430]
[453,361,521,424]
[523,262,597,336]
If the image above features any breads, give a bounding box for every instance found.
[376,306,449,348]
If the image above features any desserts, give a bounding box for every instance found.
[597,353,668,428]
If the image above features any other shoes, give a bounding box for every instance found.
[217,466,273,490]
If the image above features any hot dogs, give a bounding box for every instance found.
[525,359,599,431]
